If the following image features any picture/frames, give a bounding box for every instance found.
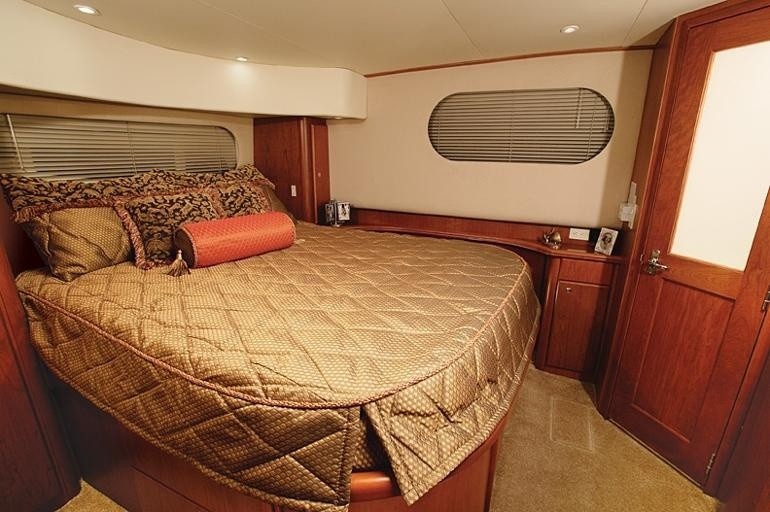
[594,226,620,256]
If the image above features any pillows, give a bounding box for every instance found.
[0,162,299,284]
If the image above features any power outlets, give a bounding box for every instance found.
[568,227,591,241]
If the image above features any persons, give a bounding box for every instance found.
[600,233,612,250]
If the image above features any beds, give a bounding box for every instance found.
[15,218,542,512]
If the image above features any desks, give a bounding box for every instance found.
[335,220,620,384]
